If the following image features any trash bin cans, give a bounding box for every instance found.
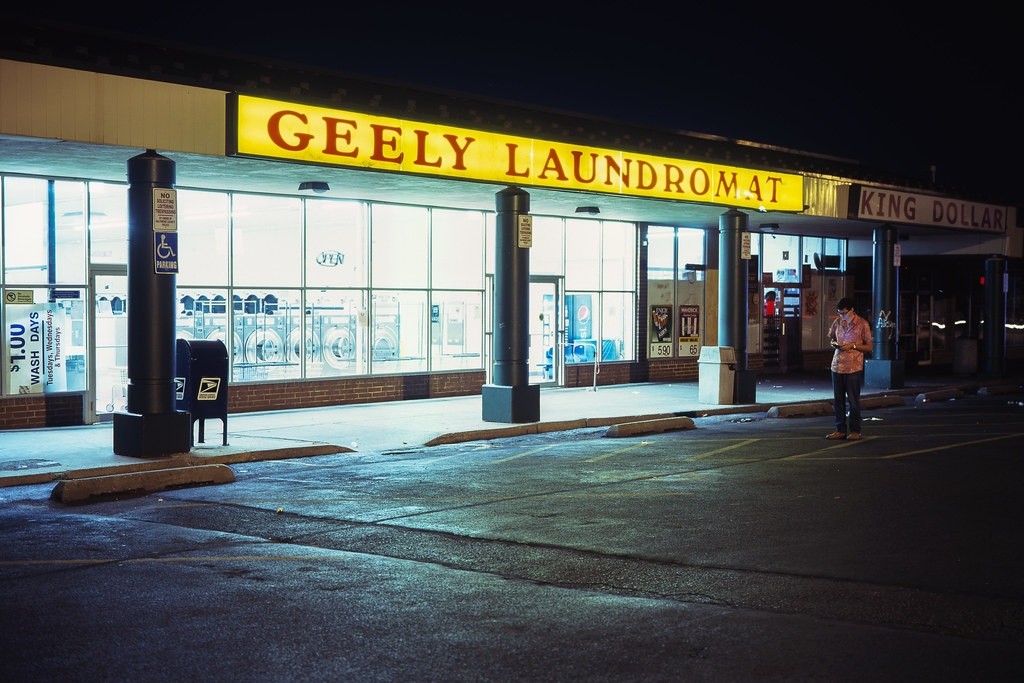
[697,345,738,406]
[176,338,233,419]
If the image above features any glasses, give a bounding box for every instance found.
[837,310,850,315]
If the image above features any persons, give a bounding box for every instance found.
[826,297,873,440]
[763,291,777,318]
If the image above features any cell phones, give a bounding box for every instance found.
[832,344,843,348]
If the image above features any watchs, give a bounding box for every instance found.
[852,343,857,349]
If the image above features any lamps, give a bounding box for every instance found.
[298,182,330,194]
[759,223,779,232]
[575,207,600,216]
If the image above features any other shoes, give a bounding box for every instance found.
[846,432,862,440]
[826,431,846,440]
